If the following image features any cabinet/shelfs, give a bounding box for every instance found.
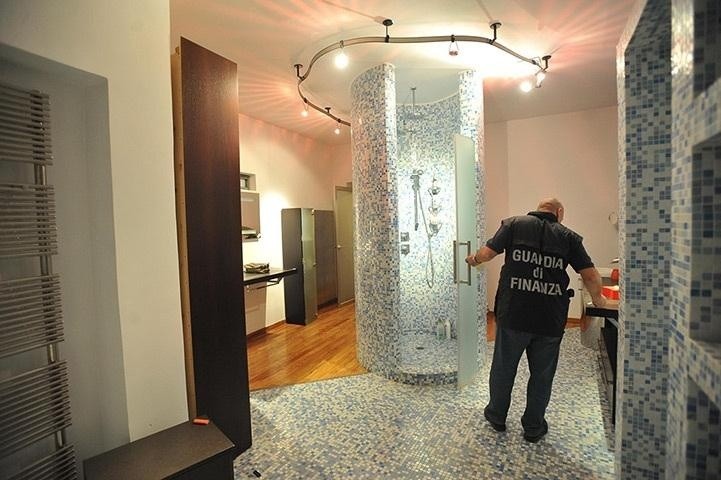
[282,209,318,326]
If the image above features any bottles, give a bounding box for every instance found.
[612,269,620,282]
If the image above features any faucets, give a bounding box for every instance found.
[609,257,619,264]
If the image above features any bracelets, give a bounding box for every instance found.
[473,256,482,265]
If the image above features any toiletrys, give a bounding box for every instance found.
[437,316,444,339]
[444,319,451,340]
[434,320,438,336]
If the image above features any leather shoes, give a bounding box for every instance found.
[525,424,547,443]
[485,405,505,431]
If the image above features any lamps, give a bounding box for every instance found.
[449,41,459,57]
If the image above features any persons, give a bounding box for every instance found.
[465,198,606,443]
